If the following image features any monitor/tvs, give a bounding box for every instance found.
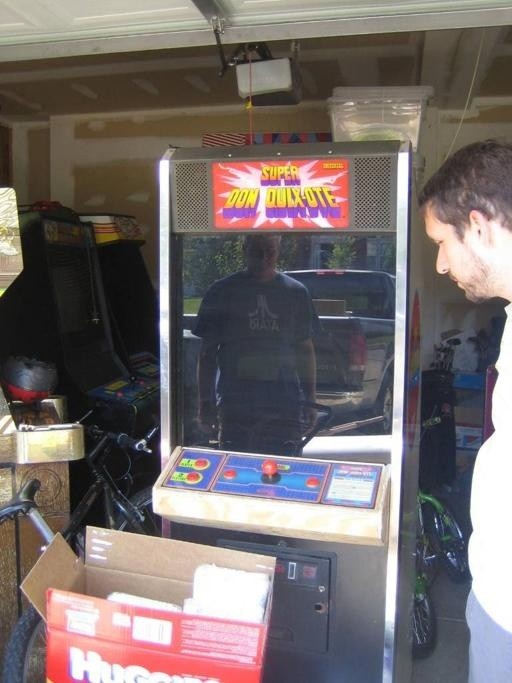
[51,248,160,357]
[170,231,396,465]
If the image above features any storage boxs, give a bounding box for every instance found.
[20,524,280,682]
[325,83,436,152]
[450,369,486,476]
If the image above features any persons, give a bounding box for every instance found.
[191,232,322,462]
[414,134,512,681]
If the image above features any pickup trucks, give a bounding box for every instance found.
[186,263,394,435]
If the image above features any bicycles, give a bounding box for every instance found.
[2,409,162,683]
[412,472,469,656]
